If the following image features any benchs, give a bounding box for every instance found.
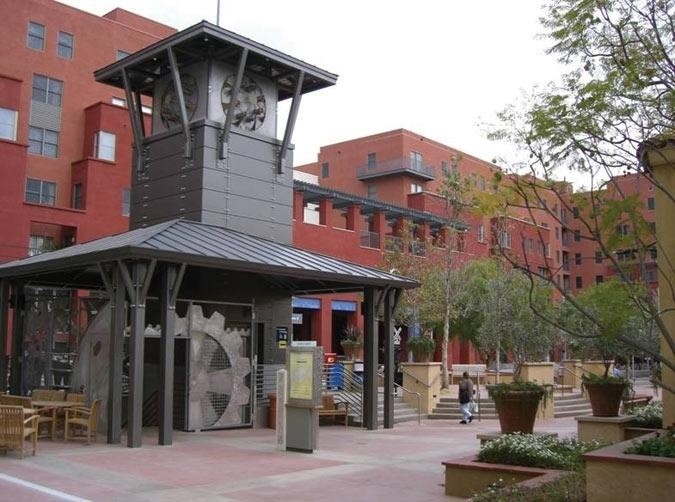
[320,394,349,427]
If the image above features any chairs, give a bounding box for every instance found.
[0,389,102,460]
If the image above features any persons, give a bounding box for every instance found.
[458,372,473,424]
[614,363,622,377]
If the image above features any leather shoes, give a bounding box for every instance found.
[459,420,466,424]
[468,415,474,423]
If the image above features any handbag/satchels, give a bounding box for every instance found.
[469,401,478,413]
[458,389,470,404]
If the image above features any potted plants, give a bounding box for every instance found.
[579,371,636,417]
[485,379,554,435]
[405,334,434,362]
